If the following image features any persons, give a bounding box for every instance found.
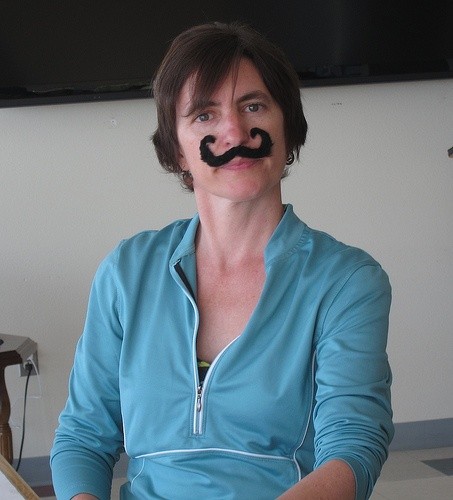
[49,18,395,500]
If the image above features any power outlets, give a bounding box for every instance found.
[19,343,39,376]
